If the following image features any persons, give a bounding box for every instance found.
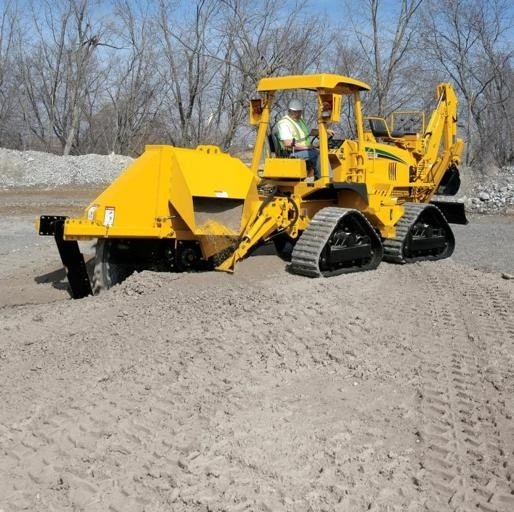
[275,98,335,181]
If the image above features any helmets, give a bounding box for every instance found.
[289,99,304,111]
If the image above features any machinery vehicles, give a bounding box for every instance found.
[35,73,469,301]
